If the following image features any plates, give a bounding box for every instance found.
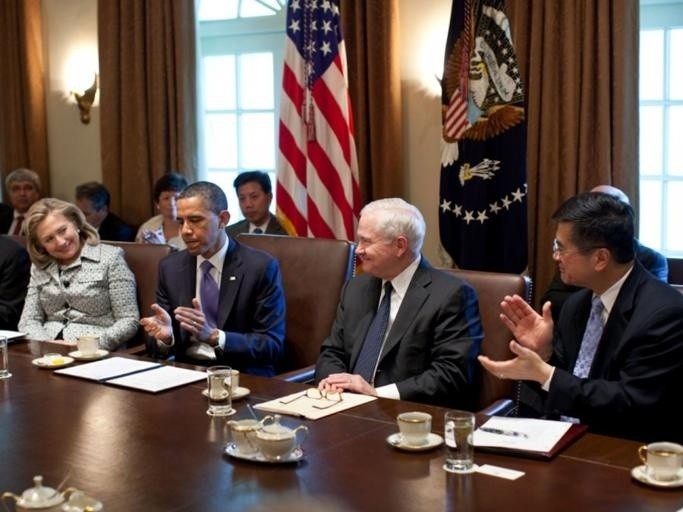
[385,432,444,449]
[30,357,73,367]
[68,349,108,360]
[223,443,303,463]
[631,466,682,487]
[202,387,250,401]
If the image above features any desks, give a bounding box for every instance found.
[0,333,683,512]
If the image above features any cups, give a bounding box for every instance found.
[231,416,272,454]
[206,366,231,414]
[0,335,8,376]
[444,411,474,469]
[638,440,682,479]
[255,415,308,457]
[396,411,431,444]
[76,337,97,355]
[214,369,239,394]
[1,475,64,511]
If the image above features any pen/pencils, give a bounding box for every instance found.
[246,404,259,421]
[480,427,527,438]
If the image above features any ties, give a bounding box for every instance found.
[561,297,604,425]
[200,261,220,328]
[353,281,393,383]
[13,216,24,235]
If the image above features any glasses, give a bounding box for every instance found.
[280,388,342,409]
[553,238,615,258]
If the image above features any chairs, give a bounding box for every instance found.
[138,232,355,389]
[95,240,180,362]
[1,234,31,260]
[264,265,534,424]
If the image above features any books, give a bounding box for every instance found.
[51,356,208,395]
[483,421,590,461]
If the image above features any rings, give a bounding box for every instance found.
[499,373,503,381]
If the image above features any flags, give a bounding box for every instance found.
[275,1,362,241]
[439,1,529,272]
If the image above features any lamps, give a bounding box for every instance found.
[63,67,98,125]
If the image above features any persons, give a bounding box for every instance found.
[476,191,682,443]
[539,185,669,325]
[316,197,483,412]
